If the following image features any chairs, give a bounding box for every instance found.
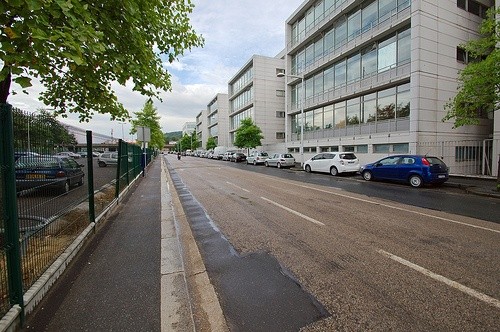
[408,158,413,164]
[343,155,348,159]
[287,155,290,158]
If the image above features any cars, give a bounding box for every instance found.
[167,149,247,163]
[302,152,361,176]
[264,152,296,169]
[14,151,134,168]
[15,154,86,195]
[245,150,270,166]
[359,154,450,188]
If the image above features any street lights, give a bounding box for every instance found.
[277,72,304,168]
